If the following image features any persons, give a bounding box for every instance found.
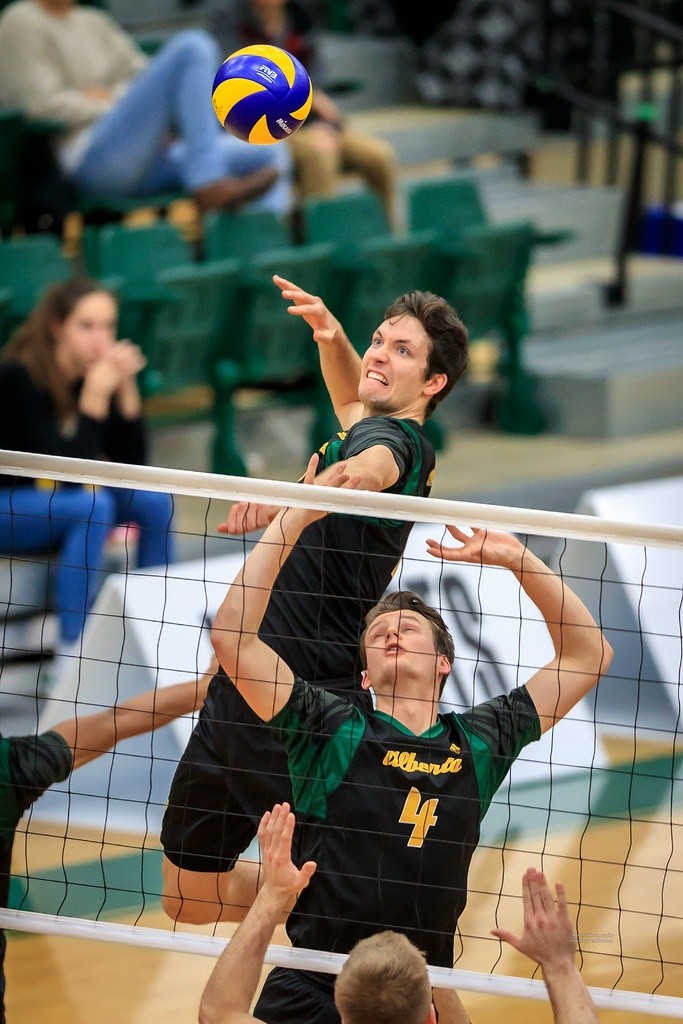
[159,272,468,931]
[0,0,293,225]
[238,0,412,240]
[1,275,175,704]
[194,802,604,1024]
[0,655,221,1023]
[210,450,613,1024]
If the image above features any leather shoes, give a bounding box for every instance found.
[199,164,280,217]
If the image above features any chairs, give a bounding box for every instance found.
[408,173,551,434]
[292,190,448,473]
[203,208,342,474]
[0,231,76,327]
[83,226,240,473]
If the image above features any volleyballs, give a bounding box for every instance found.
[214,44,312,144]
